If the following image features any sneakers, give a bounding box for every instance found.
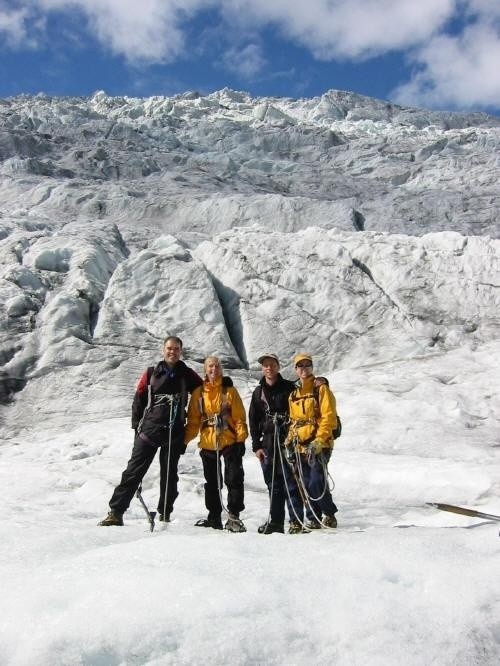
[100,508,123,525]
[208,512,222,528]
[259,521,284,533]
[289,521,302,533]
[323,515,337,528]
[160,512,170,521]
[306,519,321,529]
[226,513,244,532]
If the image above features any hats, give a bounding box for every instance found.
[257,353,279,364]
[294,353,312,364]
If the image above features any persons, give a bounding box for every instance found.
[97,336,234,526]
[284,353,339,530]
[178,356,248,533]
[248,353,330,533]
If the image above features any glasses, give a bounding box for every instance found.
[298,364,311,368]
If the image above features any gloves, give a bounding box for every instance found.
[238,442,245,456]
[181,443,186,454]
